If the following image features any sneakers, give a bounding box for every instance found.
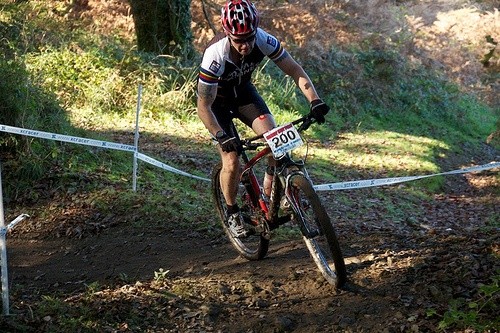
[261,189,271,203]
[224,209,250,237]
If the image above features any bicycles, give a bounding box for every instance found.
[211,112,347,289]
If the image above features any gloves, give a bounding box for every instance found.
[215,130,240,152]
[310,99,330,125]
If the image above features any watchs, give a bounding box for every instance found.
[216,131,227,140]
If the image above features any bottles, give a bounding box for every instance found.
[263,166,274,197]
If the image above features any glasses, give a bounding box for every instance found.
[227,29,258,44]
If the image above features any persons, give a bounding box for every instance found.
[198,0,330,238]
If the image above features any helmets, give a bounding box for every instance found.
[220,0,259,39]
